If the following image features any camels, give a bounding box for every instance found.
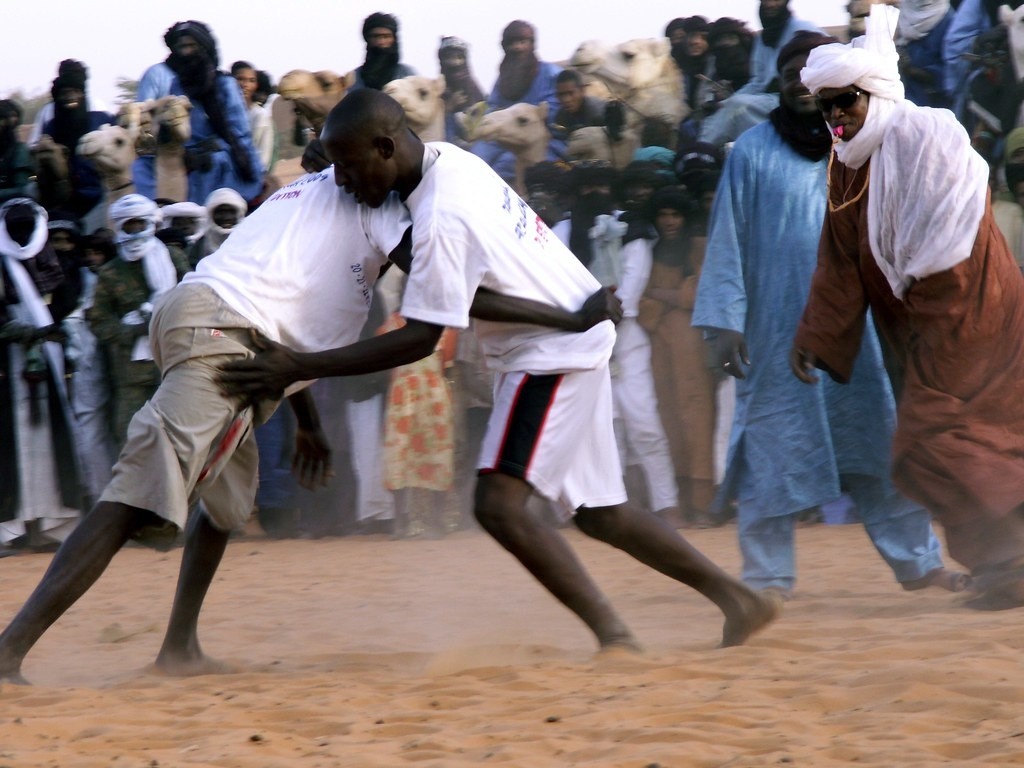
[567,36,691,153]
[453,99,643,212]
[8,90,194,242]
[259,68,447,206]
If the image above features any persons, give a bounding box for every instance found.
[690,29,974,595]
[789,32,1024,613]
[301,90,784,666]
[0,160,624,687]
[1,0,1024,555]
